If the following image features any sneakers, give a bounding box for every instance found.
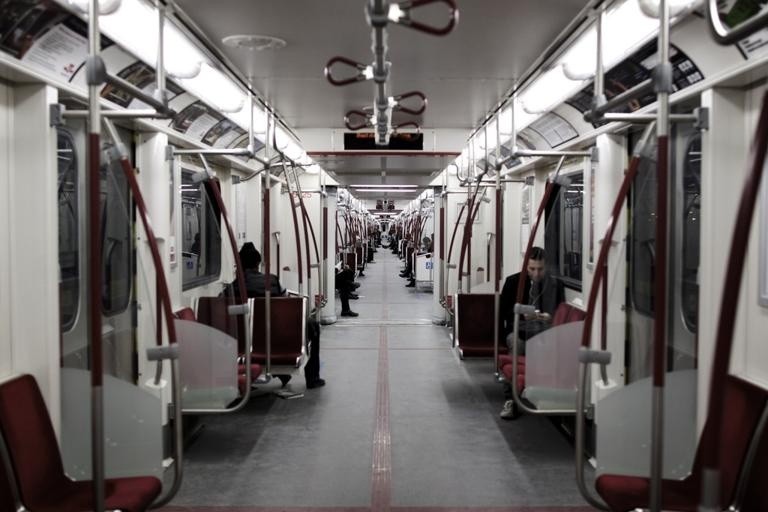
[500,400,513,417]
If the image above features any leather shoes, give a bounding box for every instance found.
[307,379,324,388]
[399,270,415,287]
[341,310,358,316]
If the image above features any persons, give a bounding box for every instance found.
[335,222,434,317]
[498,247,561,420]
[222,242,325,390]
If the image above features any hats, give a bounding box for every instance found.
[239,243,260,266]
